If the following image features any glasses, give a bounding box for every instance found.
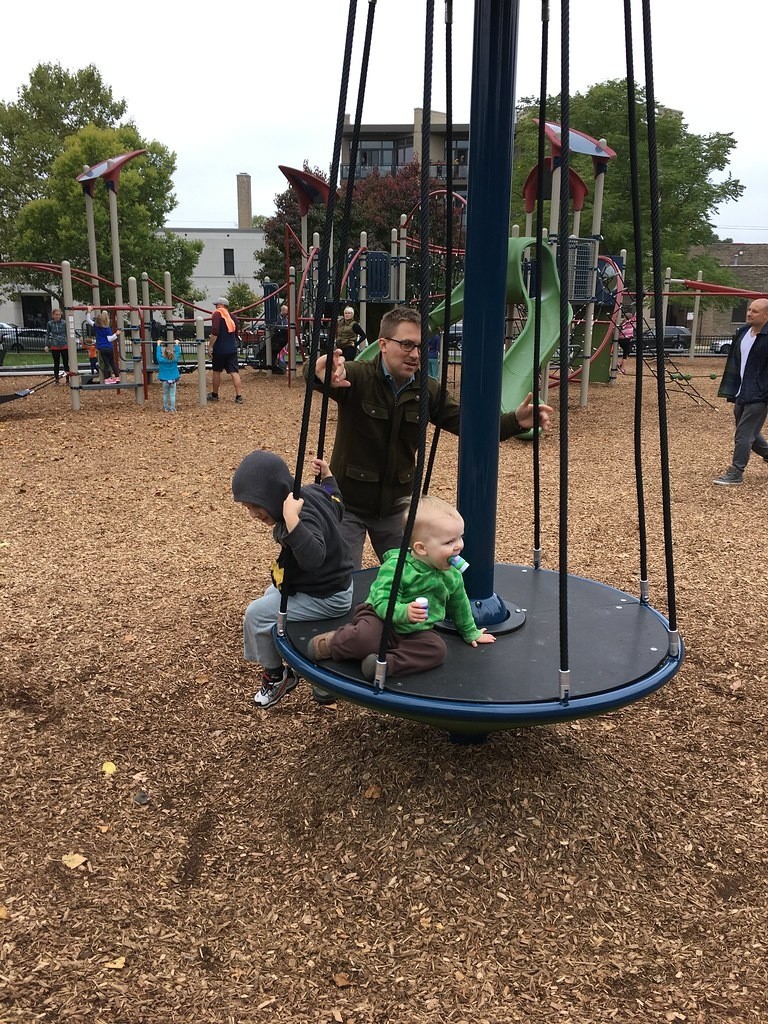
[385,337,421,354]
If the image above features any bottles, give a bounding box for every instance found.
[447,555,470,573]
[415,597,429,621]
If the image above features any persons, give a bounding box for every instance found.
[618,313,636,370]
[28,315,44,337]
[303,308,554,704]
[276,306,288,325]
[277,347,288,362]
[713,297,768,484]
[232,451,354,708]
[86,306,122,384]
[336,307,366,362]
[307,496,497,682]
[207,297,243,404]
[156,340,181,412]
[44,309,69,383]
[80,338,99,374]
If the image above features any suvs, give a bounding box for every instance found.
[236,324,266,347]
[173,326,210,340]
[440,324,462,351]
[630,326,692,353]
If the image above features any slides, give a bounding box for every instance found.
[347,235,575,440]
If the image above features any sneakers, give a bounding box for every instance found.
[713,466,743,485]
[253,667,299,709]
[115,377,120,383]
[105,378,118,384]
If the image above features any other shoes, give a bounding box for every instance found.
[54,384,58,387]
[306,630,337,661]
[362,653,378,679]
[234,397,243,403]
[66,383,70,387]
[313,686,340,705]
[206,393,219,401]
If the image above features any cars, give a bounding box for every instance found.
[0,323,83,352]
[710,340,732,354]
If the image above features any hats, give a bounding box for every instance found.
[212,297,230,307]
